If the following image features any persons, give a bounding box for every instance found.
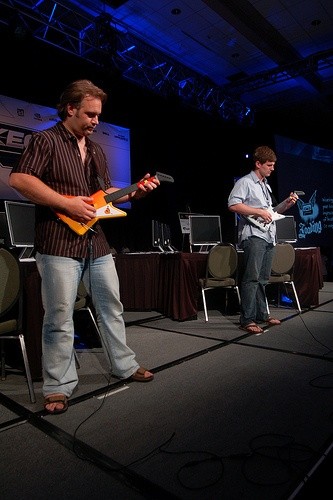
[7,79,160,414]
[227,145,299,335]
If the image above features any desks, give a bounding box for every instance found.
[287,247,324,307]
[114,252,209,322]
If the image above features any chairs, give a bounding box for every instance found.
[0,243,36,404]
[266,243,302,315]
[71,280,112,372]
[199,242,241,324]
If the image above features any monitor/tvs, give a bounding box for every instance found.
[4,200,37,260]
[276,216,297,243]
[152,215,223,254]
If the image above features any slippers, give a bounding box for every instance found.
[238,323,263,333]
[256,318,280,324]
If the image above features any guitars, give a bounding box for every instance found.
[50,172,174,235]
[241,191,305,233]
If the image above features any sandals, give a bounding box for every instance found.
[130,367,154,381]
[43,395,68,415]
